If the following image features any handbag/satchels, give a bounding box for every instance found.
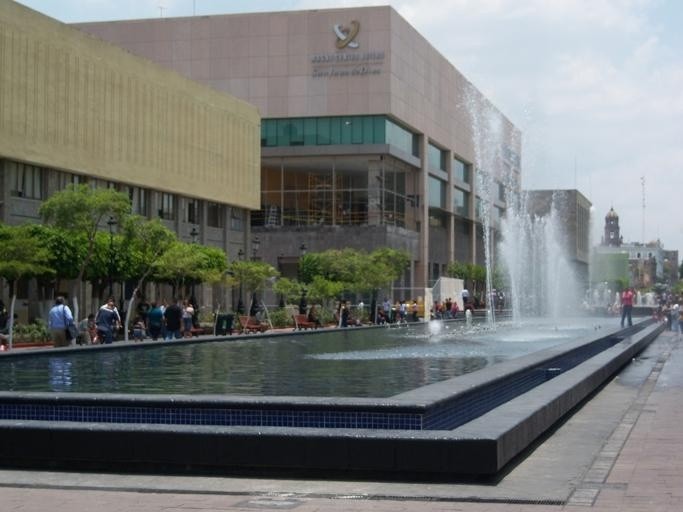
[65,323,79,340]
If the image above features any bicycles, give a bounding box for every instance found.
[92,325,122,344]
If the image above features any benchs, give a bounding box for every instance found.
[182,323,204,333]
[238,315,270,333]
[333,313,354,328]
[291,315,316,331]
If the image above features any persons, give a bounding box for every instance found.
[46,294,75,347]
[298,292,308,331]
[650,287,682,335]
[77,312,94,346]
[307,303,323,330]
[91,296,120,345]
[496,291,504,314]
[618,287,633,328]
[336,295,459,327]
[132,293,199,342]
[96,301,123,344]
